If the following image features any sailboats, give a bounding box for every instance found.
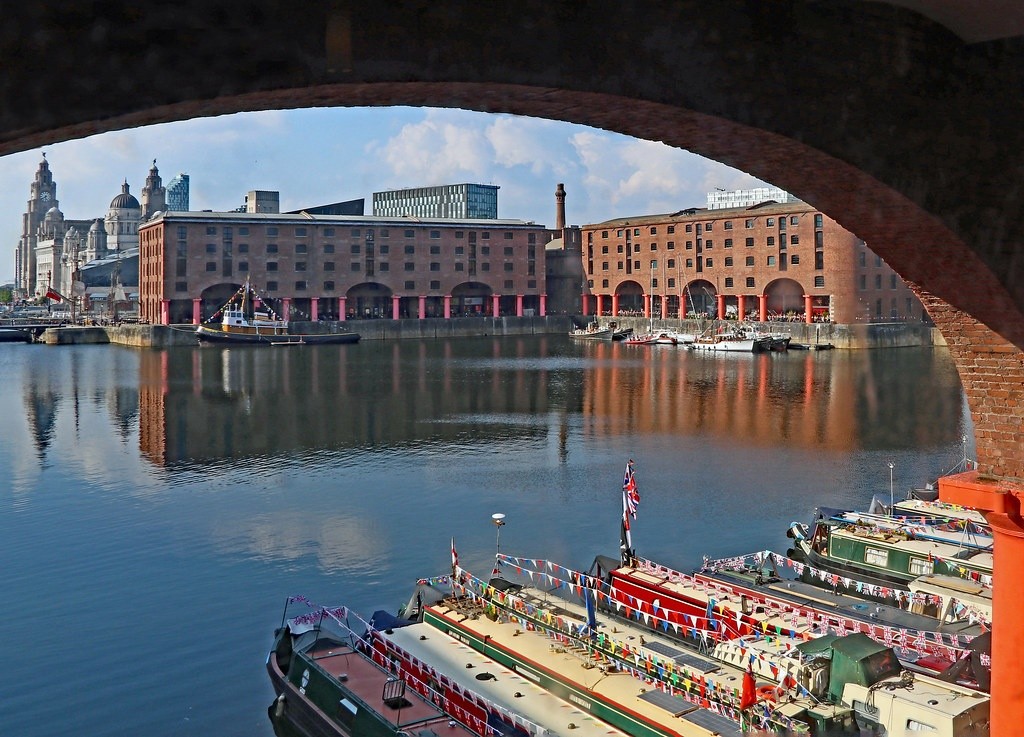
[645,250,793,353]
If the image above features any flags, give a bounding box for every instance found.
[290,595,344,626]
[638,551,991,666]
[620,492,632,567]
[204,284,282,325]
[451,542,458,566]
[623,461,640,521]
[290,306,346,331]
[740,659,757,712]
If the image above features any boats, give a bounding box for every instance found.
[268,339,309,345]
[192,274,363,344]
[602,320,634,342]
[568,319,614,340]
[265,472,992,737]
[620,335,662,345]
[786,343,811,350]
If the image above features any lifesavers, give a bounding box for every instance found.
[756,685,785,700]
[514,599,523,612]
